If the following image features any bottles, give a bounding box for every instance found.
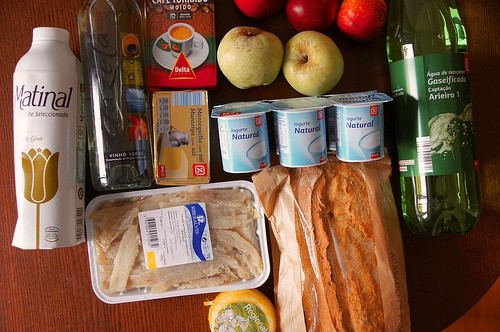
[12,27,86,250]
[386,0,480,235]
[77,0,153,191]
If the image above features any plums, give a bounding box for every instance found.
[235,0,389,42]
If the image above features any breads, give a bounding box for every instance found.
[289,152,386,332]
[208,289,277,332]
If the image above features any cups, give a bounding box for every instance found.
[211,93,393,174]
[359,129,383,159]
[246,139,268,170]
[167,23,203,58]
[308,134,327,164]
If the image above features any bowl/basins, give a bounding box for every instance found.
[86,180,271,304]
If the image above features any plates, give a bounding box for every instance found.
[152,32,210,70]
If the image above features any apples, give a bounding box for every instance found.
[282,31,344,96]
[216,26,284,90]
